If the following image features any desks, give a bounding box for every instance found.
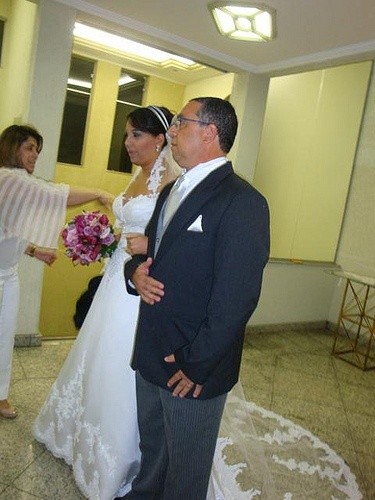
[326,268,375,372]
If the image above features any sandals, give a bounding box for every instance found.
[0,406,18,417]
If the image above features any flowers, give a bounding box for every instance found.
[61,210,118,267]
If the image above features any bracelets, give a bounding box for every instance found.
[28,245,37,257]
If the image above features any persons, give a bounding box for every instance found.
[114,96,270,500]
[0,124,118,419]
[31,105,363,500]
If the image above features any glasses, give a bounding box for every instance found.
[172,114,213,129]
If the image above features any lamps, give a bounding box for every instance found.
[206,0,277,44]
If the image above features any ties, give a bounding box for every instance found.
[163,175,184,227]
